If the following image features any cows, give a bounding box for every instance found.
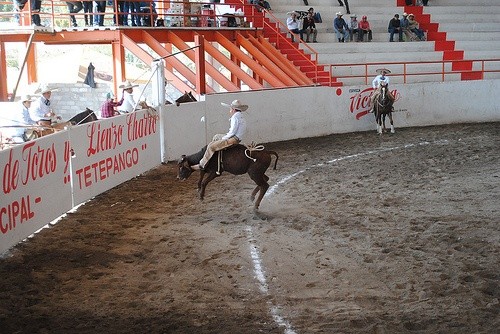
[175,142,279,208]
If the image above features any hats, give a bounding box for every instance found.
[362,15,367,19]
[402,12,408,16]
[220,99,248,111]
[19,95,34,102]
[105,92,118,100]
[119,80,139,88]
[408,13,414,16]
[37,85,53,94]
[287,10,300,17]
[336,11,344,16]
[349,13,358,18]
[394,14,399,18]
[376,67,391,75]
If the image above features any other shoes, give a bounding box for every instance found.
[190,165,206,171]
[306,40,311,43]
[389,38,396,42]
[421,36,426,41]
[399,39,405,42]
[369,108,374,112]
[409,36,418,41]
[313,41,318,43]
[337,38,344,42]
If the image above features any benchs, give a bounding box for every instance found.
[249,0,500,85]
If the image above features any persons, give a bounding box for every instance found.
[117,80,141,115]
[332,12,373,42]
[287,8,319,43]
[368,68,391,113]
[190,100,248,170]
[388,12,426,42]
[405,0,429,7]
[35,87,62,126]
[248,0,272,12]
[101,92,124,119]
[337,0,350,14]
[9,94,46,145]
[13,0,45,27]
[66,0,157,27]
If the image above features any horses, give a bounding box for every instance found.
[177,90,196,104]
[372,86,396,135]
[35,107,98,138]
